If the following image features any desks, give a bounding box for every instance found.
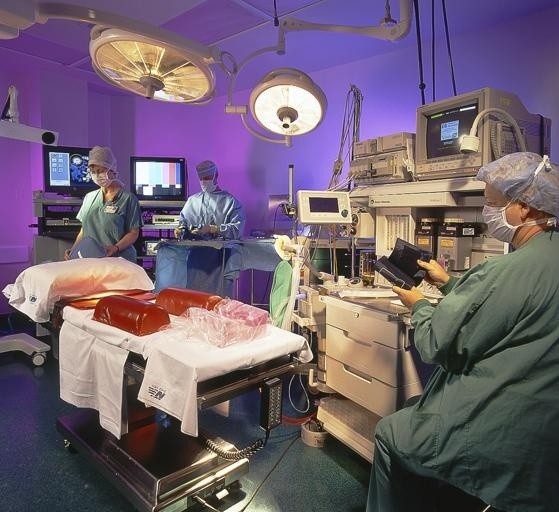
[156,238,292,312]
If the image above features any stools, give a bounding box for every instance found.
[388,472,498,511]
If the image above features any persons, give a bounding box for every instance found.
[365,151,559,512]
[63,146,144,265]
[174,160,245,299]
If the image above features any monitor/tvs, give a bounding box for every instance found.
[129,155,187,208]
[41,145,100,196]
[425,103,478,158]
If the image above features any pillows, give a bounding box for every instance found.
[3,255,156,325]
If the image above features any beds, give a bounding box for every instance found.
[2,253,315,510]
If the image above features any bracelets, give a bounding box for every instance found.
[115,245,119,256]
[208,225,217,233]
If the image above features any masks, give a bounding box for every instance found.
[200,180,216,193]
[91,172,111,188]
[482,204,518,244]
